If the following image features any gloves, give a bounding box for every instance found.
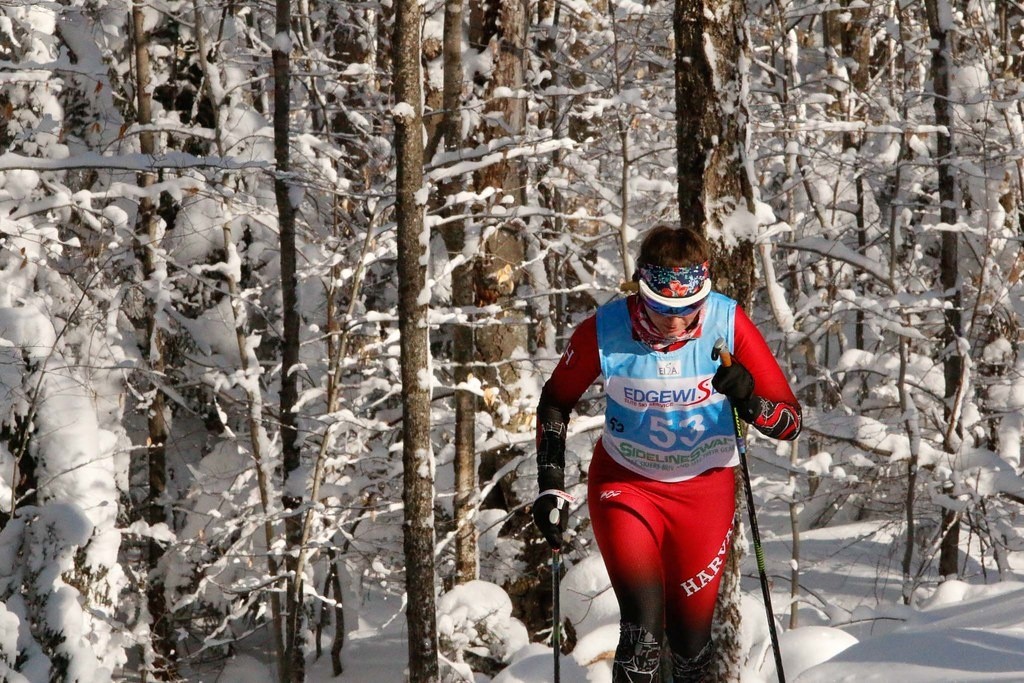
[532,471,577,550]
[712,363,755,402]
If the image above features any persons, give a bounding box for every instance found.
[533,225,803,683]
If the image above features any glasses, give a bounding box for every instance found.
[638,278,712,317]
